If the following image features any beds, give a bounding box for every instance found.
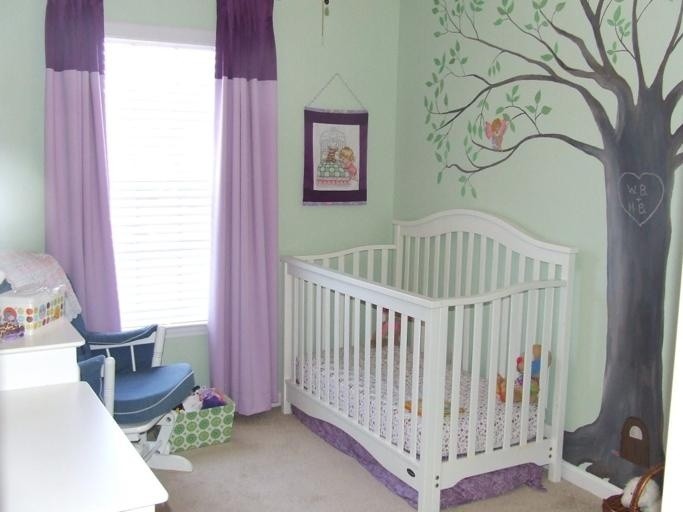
[276,210,581,511]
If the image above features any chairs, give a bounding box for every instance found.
[0,274,194,472]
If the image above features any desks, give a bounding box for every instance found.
[0,315,168,511]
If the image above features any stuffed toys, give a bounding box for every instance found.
[496,342,552,408]
[619,475,662,512]
